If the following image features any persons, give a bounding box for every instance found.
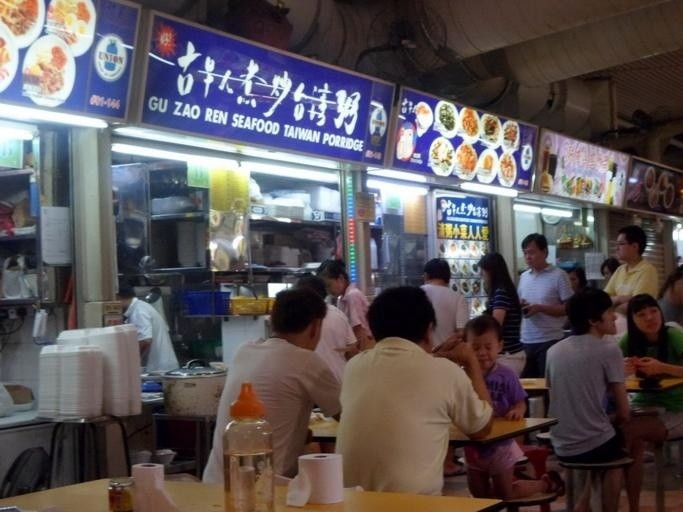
[117,282,181,371]
[337,287,494,496]
[613,291,683,512]
[297,234,575,394]
[198,288,344,487]
[548,223,682,477]
[461,313,567,498]
[545,282,631,512]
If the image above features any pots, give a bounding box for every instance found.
[161,357,228,418]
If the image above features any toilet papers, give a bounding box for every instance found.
[131,463,180,512]
[284,453,345,508]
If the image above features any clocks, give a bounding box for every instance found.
[541,213,561,224]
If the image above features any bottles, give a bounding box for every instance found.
[540,145,549,170]
[220,379,277,511]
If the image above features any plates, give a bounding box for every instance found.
[416,99,522,187]
[643,166,674,210]
[0,0,99,107]
[437,238,490,316]
[538,173,552,191]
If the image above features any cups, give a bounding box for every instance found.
[547,152,556,177]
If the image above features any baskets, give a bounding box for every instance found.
[187,291,276,315]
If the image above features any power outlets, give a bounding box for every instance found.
[8,309,36,317]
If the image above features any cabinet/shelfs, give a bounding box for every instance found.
[243,214,426,292]
[113,156,215,324]
[0,170,60,307]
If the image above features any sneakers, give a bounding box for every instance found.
[542,470,565,496]
